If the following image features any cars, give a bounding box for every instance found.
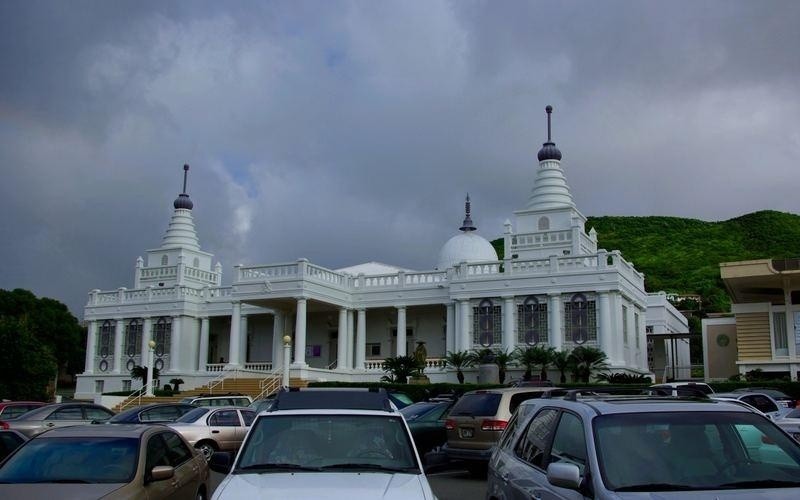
[0,430,29,466]
[165,405,259,461]
[639,381,714,425]
[509,375,555,389]
[0,402,118,439]
[0,398,61,423]
[642,392,792,456]
[106,403,202,426]
[731,388,796,410]
[0,424,210,500]
[387,389,417,409]
[757,405,799,468]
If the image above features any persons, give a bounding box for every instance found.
[413,339,427,373]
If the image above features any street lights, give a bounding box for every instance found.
[143,340,157,397]
[282,335,291,393]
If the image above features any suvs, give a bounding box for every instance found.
[178,392,257,412]
[485,386,800,500]
[400,386,461,470]
[210,385,437,500]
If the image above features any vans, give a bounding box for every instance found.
[445,387,570,477]
